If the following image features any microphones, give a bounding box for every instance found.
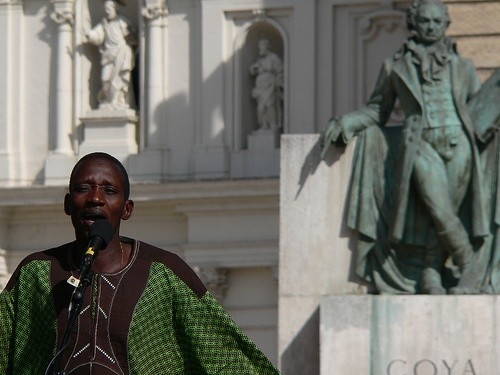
[82,219,115,266]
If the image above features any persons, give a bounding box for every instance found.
[1,148,288,374]
[244,37,284,134]
[452,43,500,295]
[78,1,139,110]
[313,0,484,298]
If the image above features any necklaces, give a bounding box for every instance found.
[70,236,124,279]
